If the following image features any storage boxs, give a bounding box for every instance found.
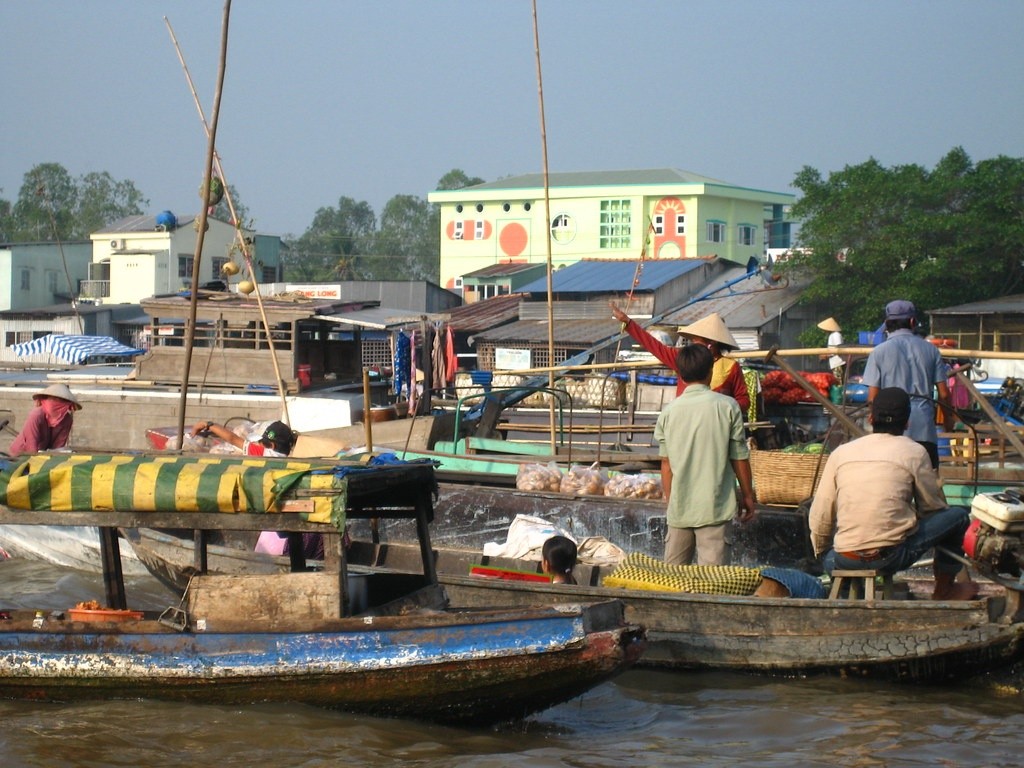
[971,492,1024,534]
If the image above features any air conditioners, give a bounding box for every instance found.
[109,240,124,250]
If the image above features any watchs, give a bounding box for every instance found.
[204,422,214,432]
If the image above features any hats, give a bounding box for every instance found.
[30,381,82,412]
[818,317,842,332]
[677,312,740,353]
[872,387,912,422]
[886,299,919,326]
[262,421,294,446]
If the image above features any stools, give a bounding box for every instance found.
[829,570,895,601]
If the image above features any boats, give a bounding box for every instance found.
[145,379,1024,562]
[115,527,1024,688]
[0,455,652,738]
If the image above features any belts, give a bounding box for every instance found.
[842,549,886,562]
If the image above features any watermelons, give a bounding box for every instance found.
[782,443,829,454]
[200,177,224,205]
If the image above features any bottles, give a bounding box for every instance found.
[0,612,13,619]
[33,611,47,621]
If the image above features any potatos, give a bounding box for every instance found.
[516,468,666,500]
[758,370,837,402]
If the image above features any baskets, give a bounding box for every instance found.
[68,607,143,623]
[746,435,833,507]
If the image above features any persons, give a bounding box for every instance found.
[808,387,980,601]
[608,302,751,412]
[189,421,298,457]
[654,344,755,565]
[2,383,83,475]
[818,317,846,384]
[861,300,956,517]
[543,535,578,585]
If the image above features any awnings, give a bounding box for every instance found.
[314,307,450,329]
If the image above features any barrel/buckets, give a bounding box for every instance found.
[94,299,104,307]
[71,301,81,308]
[362,405,398,424]
[68,609,145,622]
[936,424,952,463]
[298,364,311,387]
[347,571,375,616]
